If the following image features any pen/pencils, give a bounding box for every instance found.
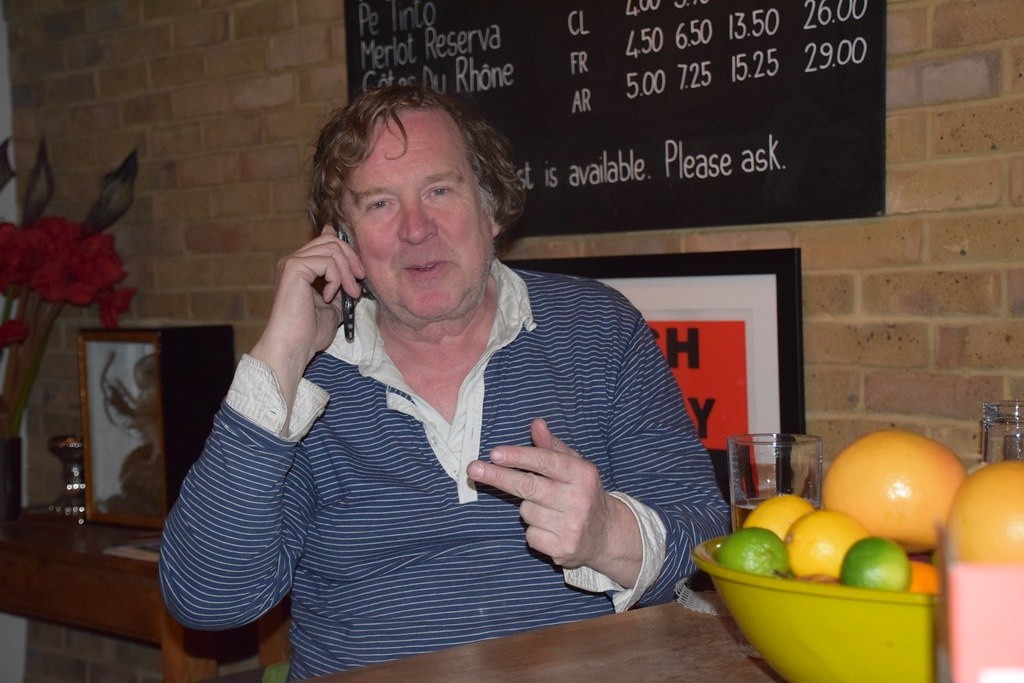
[339,229,354,343]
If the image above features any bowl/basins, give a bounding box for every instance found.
[689,534,946,683]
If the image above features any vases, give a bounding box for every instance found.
[0,438,24,515]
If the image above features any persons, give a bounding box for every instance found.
[156,84,728,683]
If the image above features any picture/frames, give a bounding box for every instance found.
[504,249,805,595]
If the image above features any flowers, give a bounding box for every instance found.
[0,134,144,434]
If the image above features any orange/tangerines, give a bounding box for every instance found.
[717,430,1024,593]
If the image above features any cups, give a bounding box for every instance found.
[729,433,825,536]
[982,399,1023,466]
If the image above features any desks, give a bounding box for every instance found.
[0,515,290,682]
[297,590,796,682]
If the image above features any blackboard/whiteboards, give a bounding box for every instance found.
[342,0,888,239]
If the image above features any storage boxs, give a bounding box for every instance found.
[75,325,234,526]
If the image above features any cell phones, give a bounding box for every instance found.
[336,228,354,343]
